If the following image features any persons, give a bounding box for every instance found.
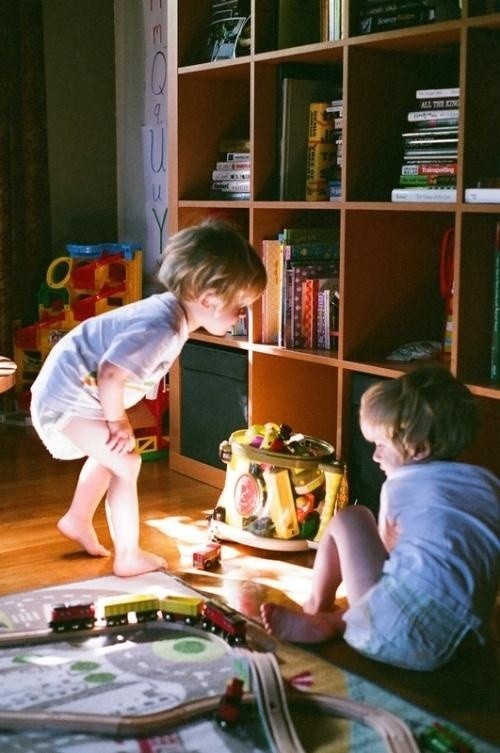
[28,216,269,577]
[212,22,227,62]
[258,365,500,675]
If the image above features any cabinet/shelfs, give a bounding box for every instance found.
[166,0,499,492]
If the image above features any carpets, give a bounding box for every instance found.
[0,569,500,753]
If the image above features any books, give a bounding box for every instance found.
[348,0,439,38]
[487,222,499,389]
[389,86,460,204]
[275,62,344,202]
[442,276,454,353]
[464,175,499,204]
[318,0,342,43]
[228,223,340,350]
[204,135,251,202]
